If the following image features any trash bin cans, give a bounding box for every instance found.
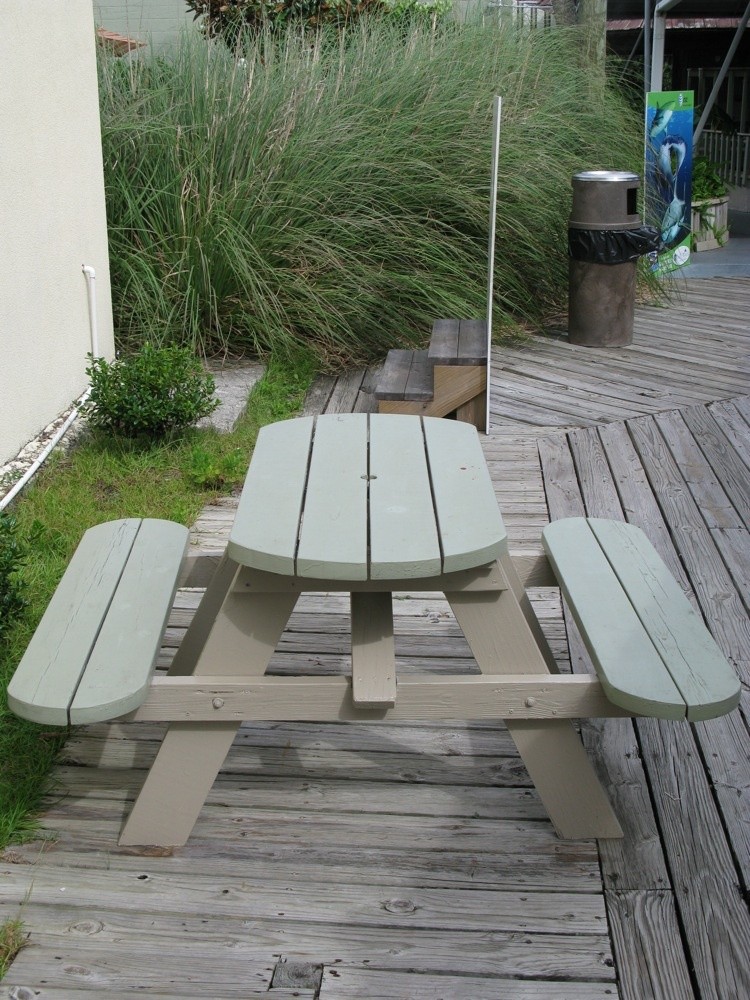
[568,171,639,347]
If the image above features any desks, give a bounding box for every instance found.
[120,413,624,847]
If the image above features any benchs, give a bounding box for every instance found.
[7,518,740,847]
